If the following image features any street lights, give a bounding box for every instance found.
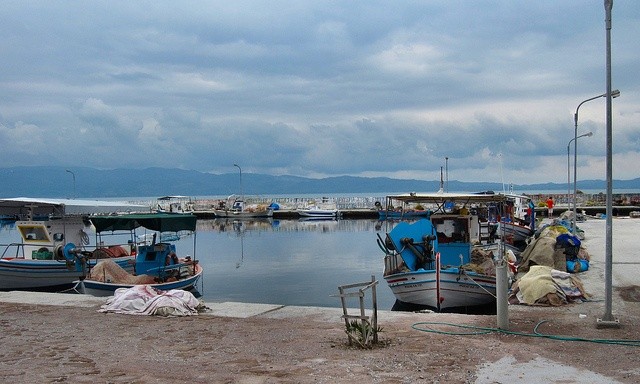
[567,131,593,211]
[572,89,621,235]
[445,154,449,193]
[234,163,243,199]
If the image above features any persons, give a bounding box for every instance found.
[545,196,555,217]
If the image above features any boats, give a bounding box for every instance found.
[380,200,433,222]
[151,196,194,214]
[0,197,152,291]
[83,215,203,301]
[382,193,513,312]
[211,204,273,217]
[482,194,535,246]
[298,200,340,220]
